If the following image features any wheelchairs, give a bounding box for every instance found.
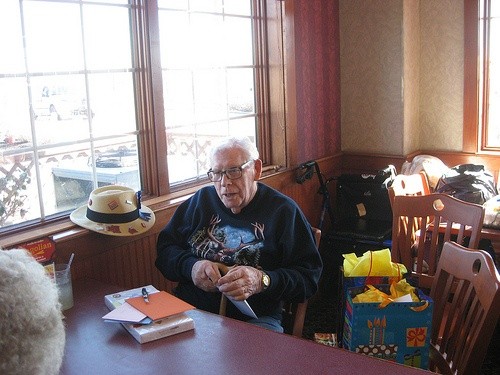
[296,160,398,267]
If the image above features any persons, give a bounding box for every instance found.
[0,247,67,375]
[154,135,323,333]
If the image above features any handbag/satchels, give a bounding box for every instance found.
[345,281,434,370]
[434,164,499,205]
[337,248,408,349]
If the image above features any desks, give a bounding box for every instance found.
[56,278,438,375]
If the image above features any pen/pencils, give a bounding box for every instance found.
[142,288,152,305]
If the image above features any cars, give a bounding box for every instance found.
[27,89,95,120]
[95,159,122,168]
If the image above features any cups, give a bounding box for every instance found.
[47,263,74,310]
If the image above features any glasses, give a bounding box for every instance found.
[207,161,254,182]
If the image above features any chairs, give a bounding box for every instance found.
[390,195,485,297]
[388,172,438,261]
[426,242,500,375]
[282,225,322,338]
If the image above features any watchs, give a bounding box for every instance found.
[260,271,270,292]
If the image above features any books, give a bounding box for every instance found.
[104,285,194,344]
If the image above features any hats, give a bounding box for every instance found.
[70,185,155,236]
